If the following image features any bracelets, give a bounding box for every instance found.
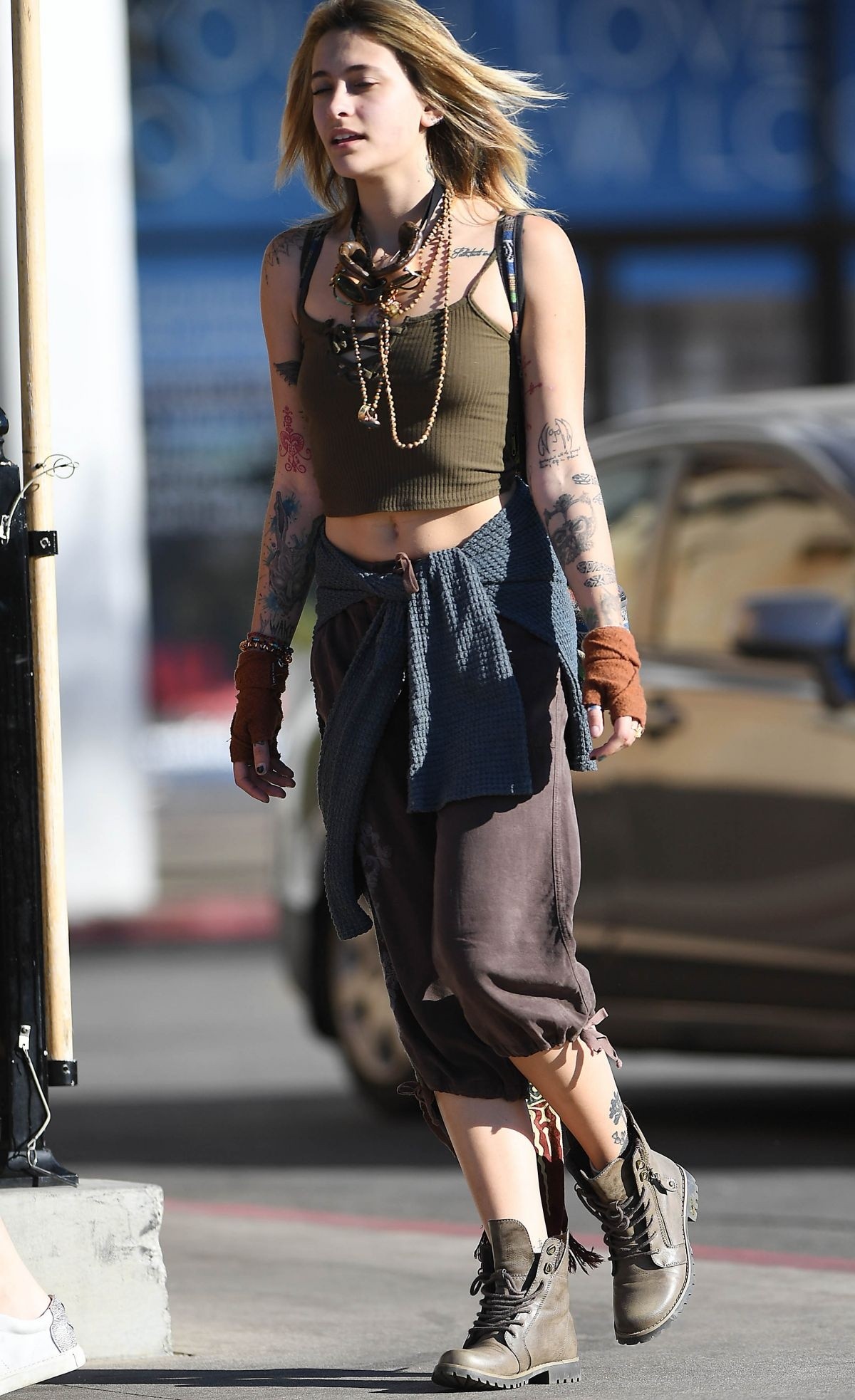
[240,632,295,665]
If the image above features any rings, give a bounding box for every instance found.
[246,764,251,767]
[632,722,643,739]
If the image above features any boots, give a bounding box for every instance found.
[564,1102,698,1345]
[431,1219,581,1391]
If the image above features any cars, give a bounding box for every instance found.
[273,384,855,1121]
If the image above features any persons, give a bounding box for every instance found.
[229,0,708,1394]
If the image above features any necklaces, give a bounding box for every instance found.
[337,174,454,450]
